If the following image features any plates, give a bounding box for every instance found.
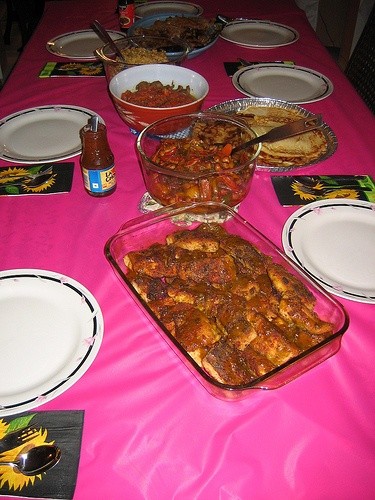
[104,200,350,402]
[0,269,104,418]
[207,98,338,172]
[220,19,300,50]
[0,105,106,164]
[135,1,203,19]
[45,29,127,62]
[232,63,334,104]
[281,199,375,305]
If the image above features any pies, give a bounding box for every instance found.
[226,107,328,166]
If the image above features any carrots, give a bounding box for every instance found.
[165,143,238,201]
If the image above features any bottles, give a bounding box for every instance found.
[117,0,135,32]
[81,124,117,198]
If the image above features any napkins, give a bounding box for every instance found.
[0,162,75,198]
[38,61,106,77]
[271,174,375,207]
[223,61,295,78]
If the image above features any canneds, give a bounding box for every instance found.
[80,123,117,198]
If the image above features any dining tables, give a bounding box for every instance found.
[0,0,375,500]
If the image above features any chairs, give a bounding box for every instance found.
[344,3,375,115]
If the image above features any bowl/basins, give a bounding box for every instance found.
[95,35,191,85]
[127,12,220,60]
[137,111,262,215]
[108,64,209,133]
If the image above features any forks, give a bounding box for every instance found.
[0,422,39,454]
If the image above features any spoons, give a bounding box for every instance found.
[0,445,62,476]
